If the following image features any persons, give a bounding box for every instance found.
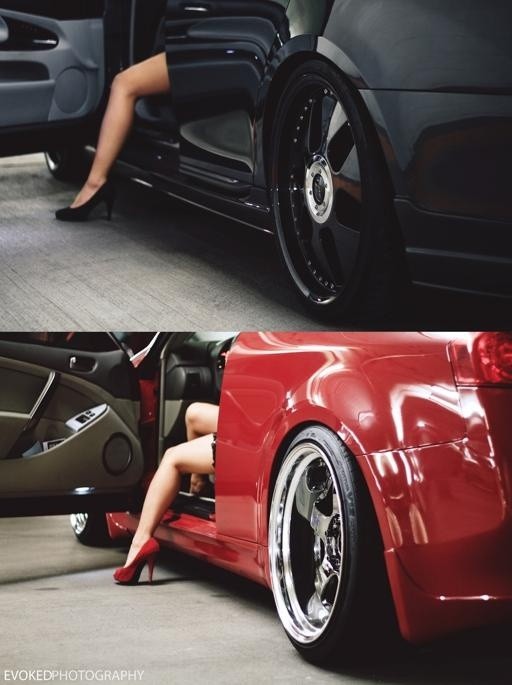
[111,400,221,587]
[54,48,256,221]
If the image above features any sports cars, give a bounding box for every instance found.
[0,333,511,682]
[1,5,507,332]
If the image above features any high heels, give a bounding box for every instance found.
[113,537,160,584]
[55,182,114,220]
